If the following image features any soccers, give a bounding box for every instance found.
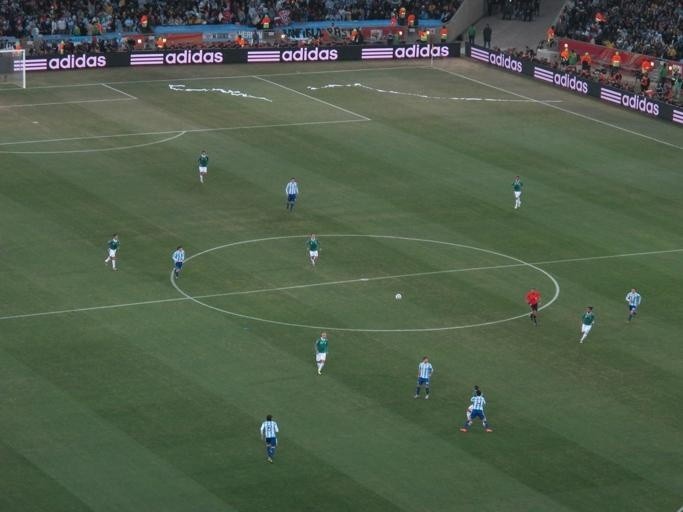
[396,293,402,299]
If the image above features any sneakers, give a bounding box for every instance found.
[580,340,584,344]
[514,201,521,209]
[485,428,493,432]
[627,312,637,323]
[267,457,272,463]
[104,260,117,271]
[317,369,322,375]
[413,394,429,399]
[286,206,292,212]
[459,427,467,432]
[530,317,536,322]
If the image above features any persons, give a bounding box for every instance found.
[304,232,320,273]
[282,176,299,213]
[523,287,541,326]
[458,389,493,433]
[509,175,523,209]
[311,329,329,376]
[464,384,479,420]
[195,149,209,185]
[468,1,682,108]
[1,1,462,56]
[411,354,434,400]
[169,245,184,279]
[624,287,641,324]
[258,413,280,463]
[577,305,596,345]
[103,232,120,271]
